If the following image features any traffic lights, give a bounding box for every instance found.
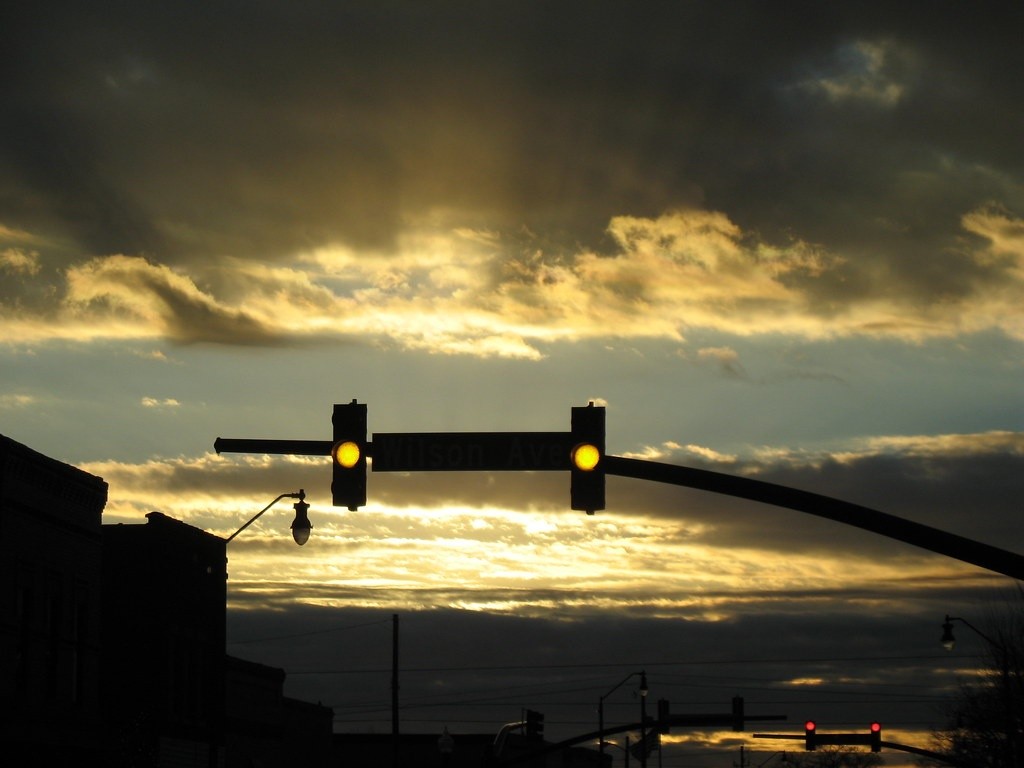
[870,723,882,752]
[805,720,816,751]
[568,406,607,510]
[528,713,547,749]
[331,404,368,511]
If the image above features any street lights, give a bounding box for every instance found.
[940,616,1010,768]
[595,674,650,768]
[437,727,453,768]
[205,489,314,768]
